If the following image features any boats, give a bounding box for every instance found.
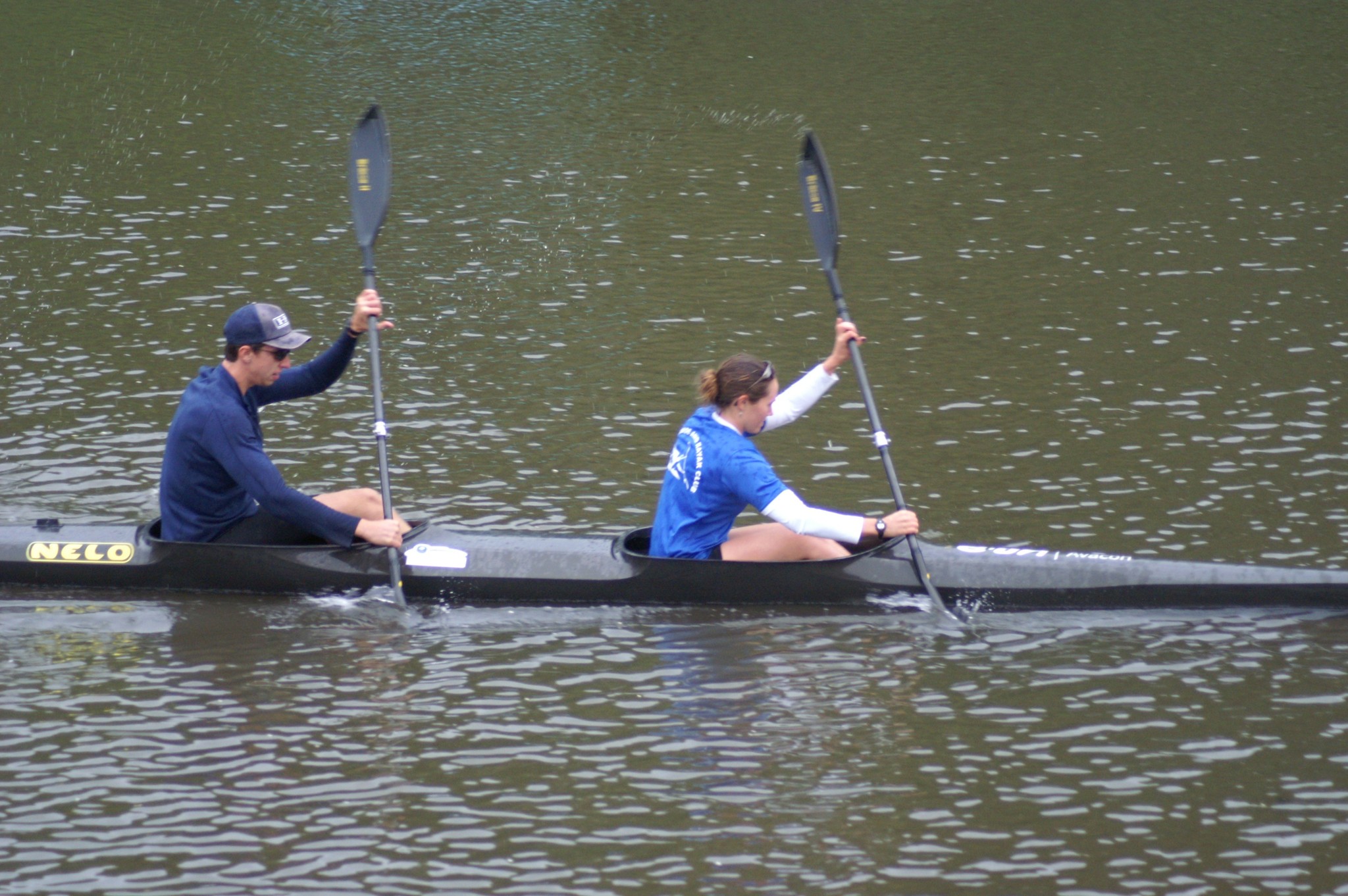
[0,516,1348,605]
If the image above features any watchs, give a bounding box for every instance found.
[875,516,887,540]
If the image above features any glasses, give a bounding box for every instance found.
[734,360,775,406]
[260,349,290,361]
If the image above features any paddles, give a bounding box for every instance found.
[344,101,414,621]
[796,128,970,630]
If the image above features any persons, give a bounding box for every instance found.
[158,289,412,549]
[650,318,919,562]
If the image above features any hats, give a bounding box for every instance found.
[223,301,313,349]
